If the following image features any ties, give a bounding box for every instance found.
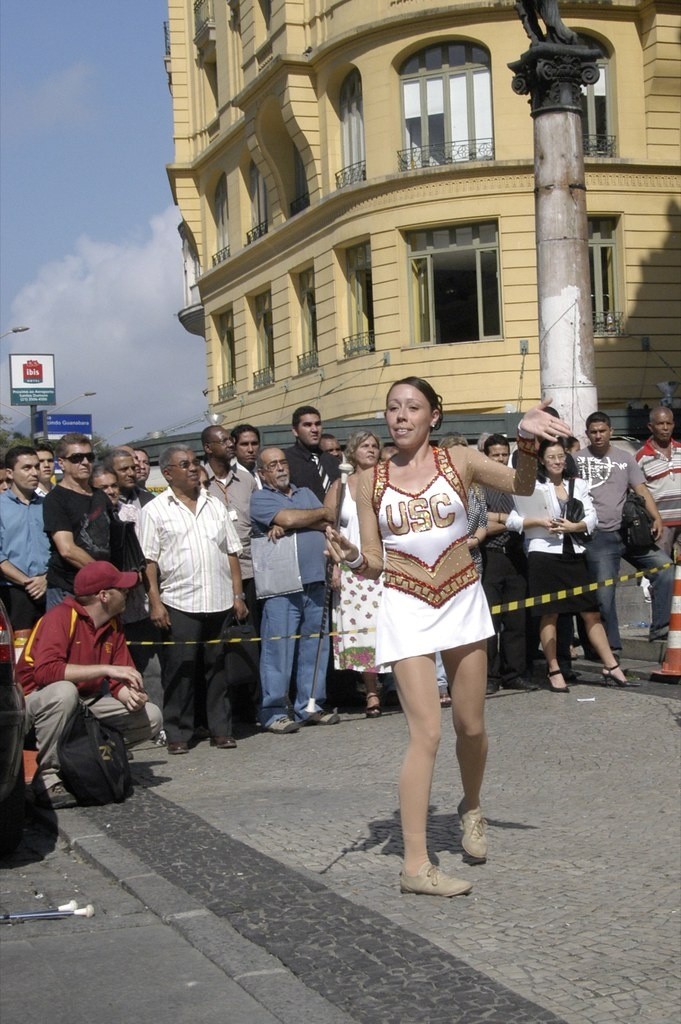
[312,452,331,495]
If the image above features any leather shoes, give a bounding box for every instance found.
[165,740,188,753]
[209,734,237,749]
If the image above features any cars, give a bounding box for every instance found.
[0,600,27,854]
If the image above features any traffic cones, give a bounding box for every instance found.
[649,553,681,685]
[14,629,39,784]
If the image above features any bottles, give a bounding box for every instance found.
[13,629,32,667]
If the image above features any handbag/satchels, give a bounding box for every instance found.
[622,492,656,555]
[111,545,152,624]
[57,702,134,807]
[562,475,592,545]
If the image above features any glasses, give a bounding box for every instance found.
[166,457,201,469]
[207,436,236,444]
[61,452,96,464]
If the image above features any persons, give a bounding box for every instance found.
[435,407,581,707]
[16,561,163,809]
[634,407,681,557]
[322,376,572,897]
[504,438,641,693]
[0,406,398,755]
[572,412,675,663]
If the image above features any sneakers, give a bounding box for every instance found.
[268,716,299,734]
[399,862,473,897]
[33,782,78,810]
[459,796,488,859]
[297,710,340,726]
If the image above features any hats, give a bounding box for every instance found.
[74,560,138,596]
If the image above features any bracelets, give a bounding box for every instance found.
[344,553,363,569]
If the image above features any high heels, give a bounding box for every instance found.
[602,664,642,687]
[546,669,570,693]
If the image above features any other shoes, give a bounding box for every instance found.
[570,644,579,660]
[437,645,548,708]
[366,695,381,717]
[645,596,651,604]
[612,653,620,662]
[653,632,670,639]
[155,730,167,746]
